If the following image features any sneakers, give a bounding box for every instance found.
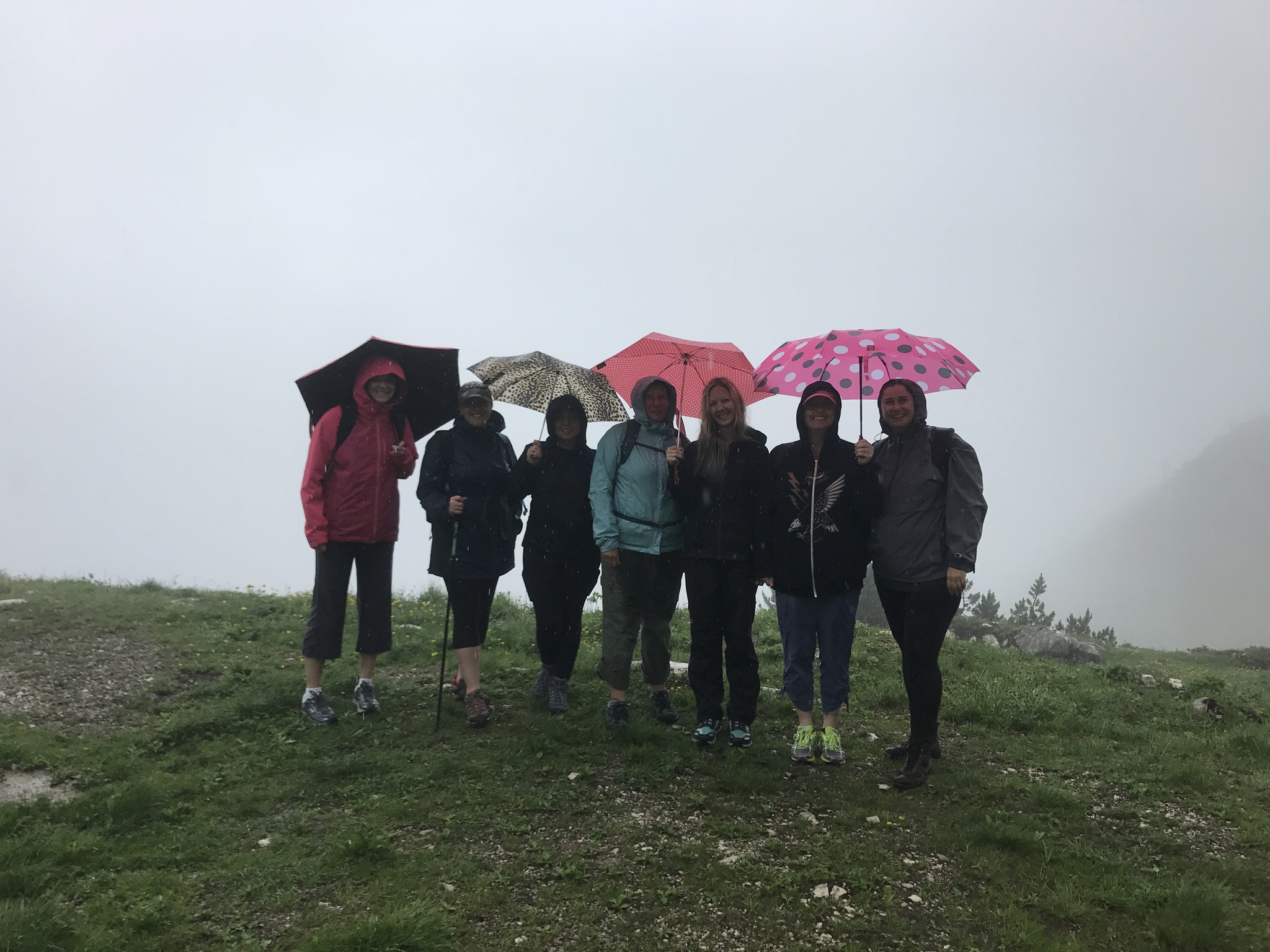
[894,744,934,787]
[691,717,722,744]
[606,697,630,730]
[819,725,846,765]
[302,688,337,725]
[884,738,942,757]
[465,688,491,724]
[728,717,752,748]
[790,723,817,762]
[353,680,381,713]
[452,675,465,699]
[650,690,679,724]
[546,677,570,712]
[534,666,551,696]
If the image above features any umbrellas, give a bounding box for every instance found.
[294,336,461,442]
[590,331,776,485]
[466,352,631,448]
[751,329,982,442]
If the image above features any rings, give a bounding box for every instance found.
[673,457,674,462]
[605,560,608,562]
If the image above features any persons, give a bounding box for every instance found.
[587,375,691,733]
[765,381,867,765]
[301,355,419,728]
[665,378,775,748]
[503,394,602,713]
[416,383,527,728]
[865,379,988,789]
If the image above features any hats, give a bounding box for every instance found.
[459,382,492,403]
[801,390,837,404]
